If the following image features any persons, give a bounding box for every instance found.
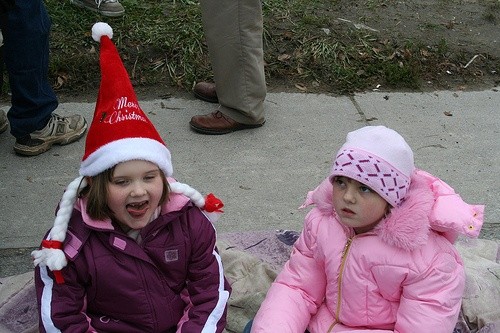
[31,22,231,333]
[190,0,267,134]
[0,0,88,157]
[244,124,485,333]
[70,0,125,17]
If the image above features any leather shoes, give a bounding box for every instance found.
[193,82,219,104]
[191,112,266,134]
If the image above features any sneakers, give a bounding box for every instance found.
[14,113,88,156]
[70,0,125,16]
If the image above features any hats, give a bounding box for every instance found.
[329,126,414,207]
[30,22,224,284]
